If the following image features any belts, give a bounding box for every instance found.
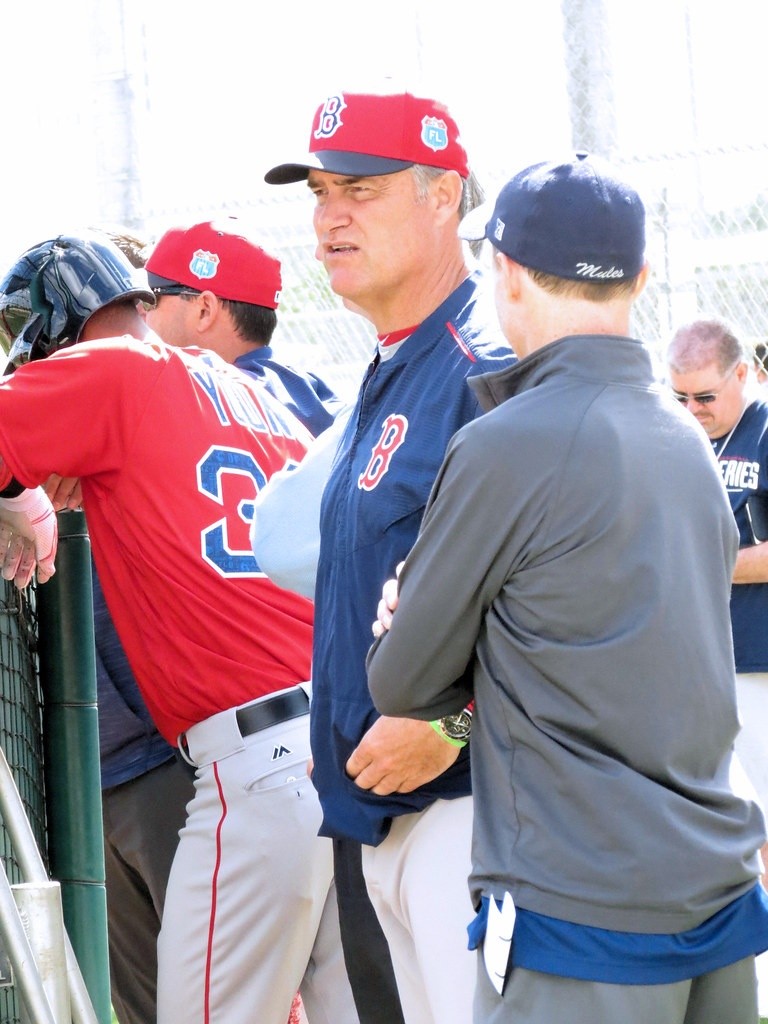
[173,687,310,773]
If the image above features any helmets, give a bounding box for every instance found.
[0,231,157,376]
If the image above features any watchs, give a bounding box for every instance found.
[436,709,473,744]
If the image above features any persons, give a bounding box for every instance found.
[365,148,768,1024]
[0,221,402,1024]
[665,321,768,889]
[262,88,521,1024]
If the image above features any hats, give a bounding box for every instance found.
[457,150,646,280]
[144,220,282,310]
[265,90,470,184]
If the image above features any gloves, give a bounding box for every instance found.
[0,485,59,589]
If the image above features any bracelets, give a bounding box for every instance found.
[429,719,467,747]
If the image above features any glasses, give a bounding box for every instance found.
[142,286,202,311]
[674,357,744,405]
